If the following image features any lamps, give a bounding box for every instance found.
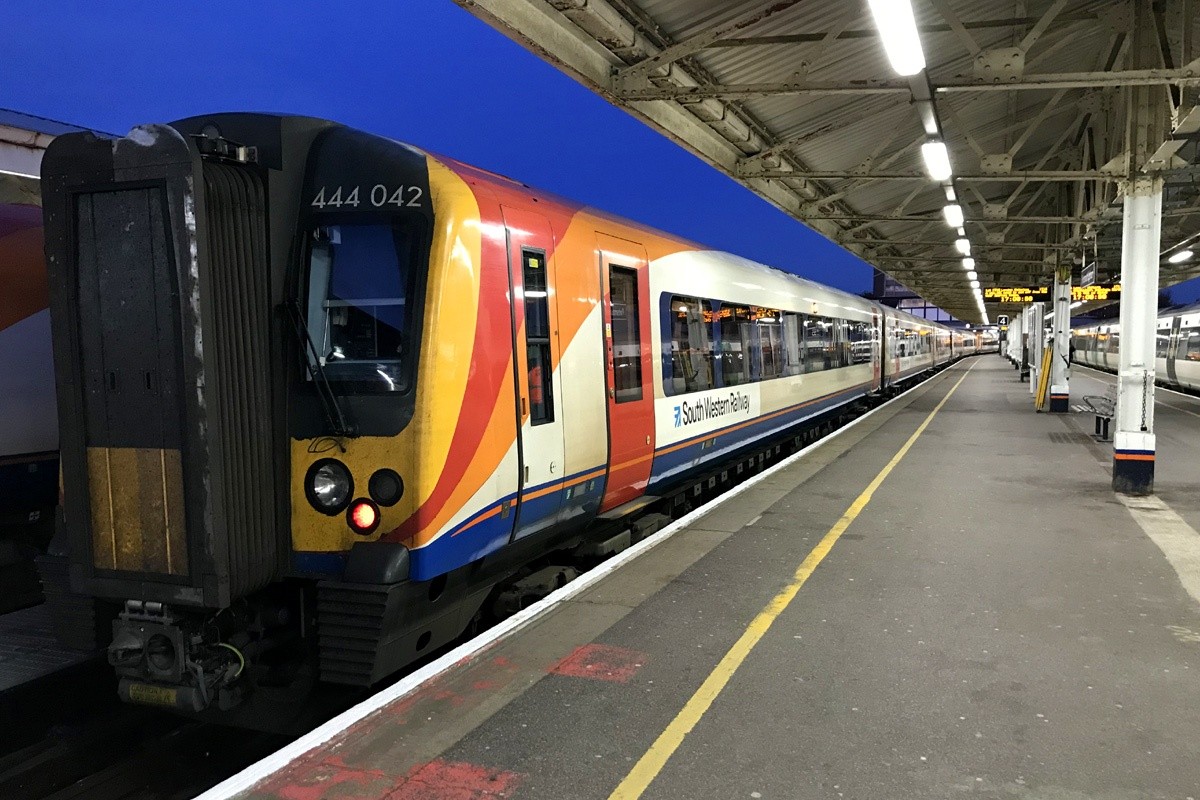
[866,0,975,270]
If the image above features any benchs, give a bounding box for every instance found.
[1020,368,1029,381]
[1080,383,1119,440]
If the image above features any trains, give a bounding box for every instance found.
[40,113,999,715]
[1072,313,1200,392]
